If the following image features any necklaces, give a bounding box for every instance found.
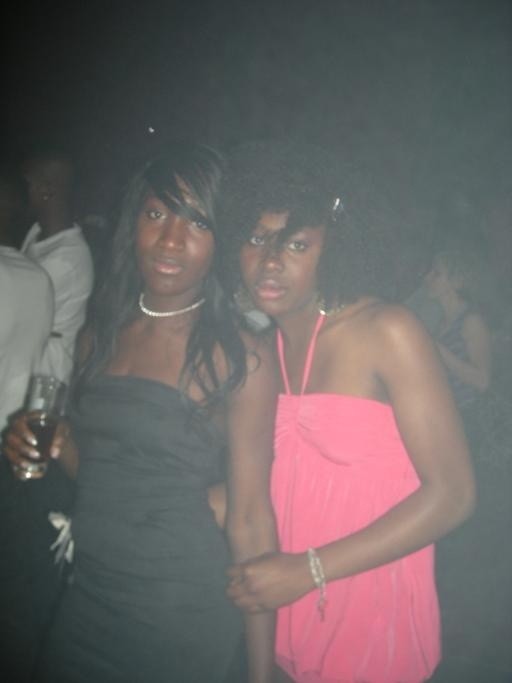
[138,292,206,318]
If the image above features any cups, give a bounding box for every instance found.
[9,376,67,484]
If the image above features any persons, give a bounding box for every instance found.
[223,183,475,683]
[416,257,491,414]
[1,138,280,683]
[1,147,96,682]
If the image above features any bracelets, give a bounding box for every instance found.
[308,547,325,587]
